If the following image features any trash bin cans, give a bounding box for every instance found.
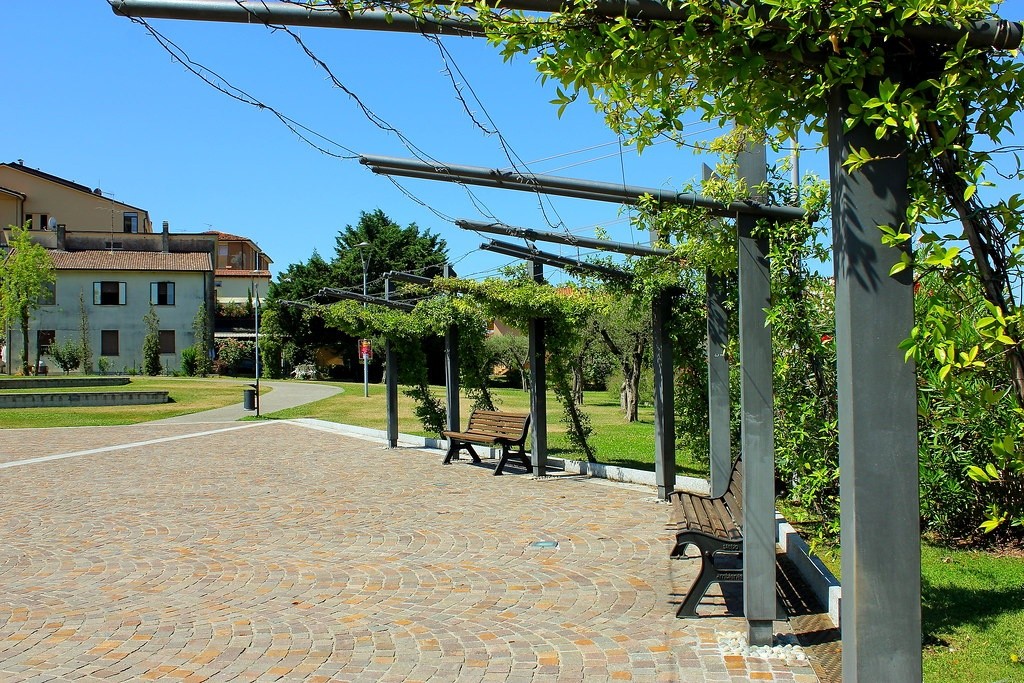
[243,383,257,412]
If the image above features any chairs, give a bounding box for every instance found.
[32,365,49,375]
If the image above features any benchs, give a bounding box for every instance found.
[667,449,759,621]
[440,408,532,476]
